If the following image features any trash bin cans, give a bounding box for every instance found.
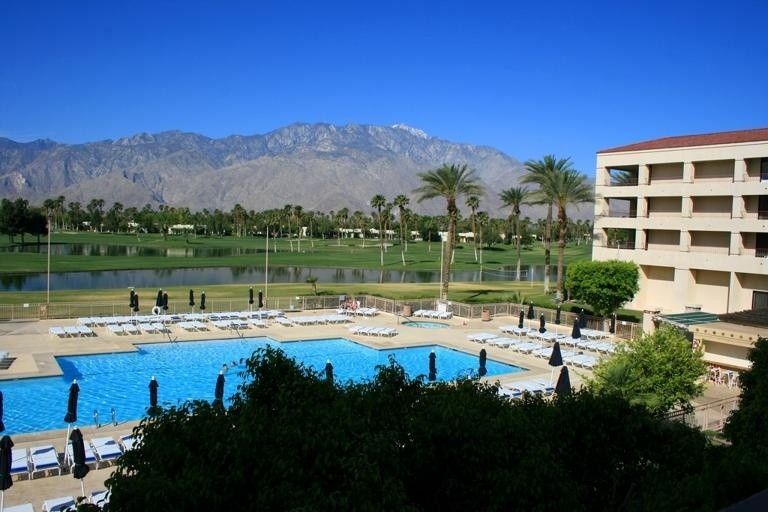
[403,304,412,316]
[39,303,49,320]
[482,309,490,321]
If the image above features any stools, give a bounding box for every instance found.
[0,351,10,365]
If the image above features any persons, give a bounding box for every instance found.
[339,297,358,311]
[230,361,235,366]
[223,363,227,370]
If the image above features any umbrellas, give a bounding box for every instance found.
[200,291,205,324]
[129,288,134,324]
[162,290,168,326]
[0,435,14,512]
[147,376,162,415]
[133,292,139,326]
[478,346,487,376]
[428,350,437,381]
[212,370,225,409]
[555,366,572,398]
[69,427,90,504]
[63,379,80,465]
[325,359,333,384]
[156,288,164,324]
[248,287,253,319]
[548,340,563,387]
[258,289,263,320]
[518,301,616,352]
[189,290,195,322]
[0,390,4,432]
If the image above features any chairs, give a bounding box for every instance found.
[464,319,739,402]
[275,314,354,329]
[176,321,210,333]
[336,305,380,318]
[412,308,454,320]
[349,324,401,340]
[48,325,94,339]
[75,310,286,329]
[104,322,171,337]
[1,432,147,512]
